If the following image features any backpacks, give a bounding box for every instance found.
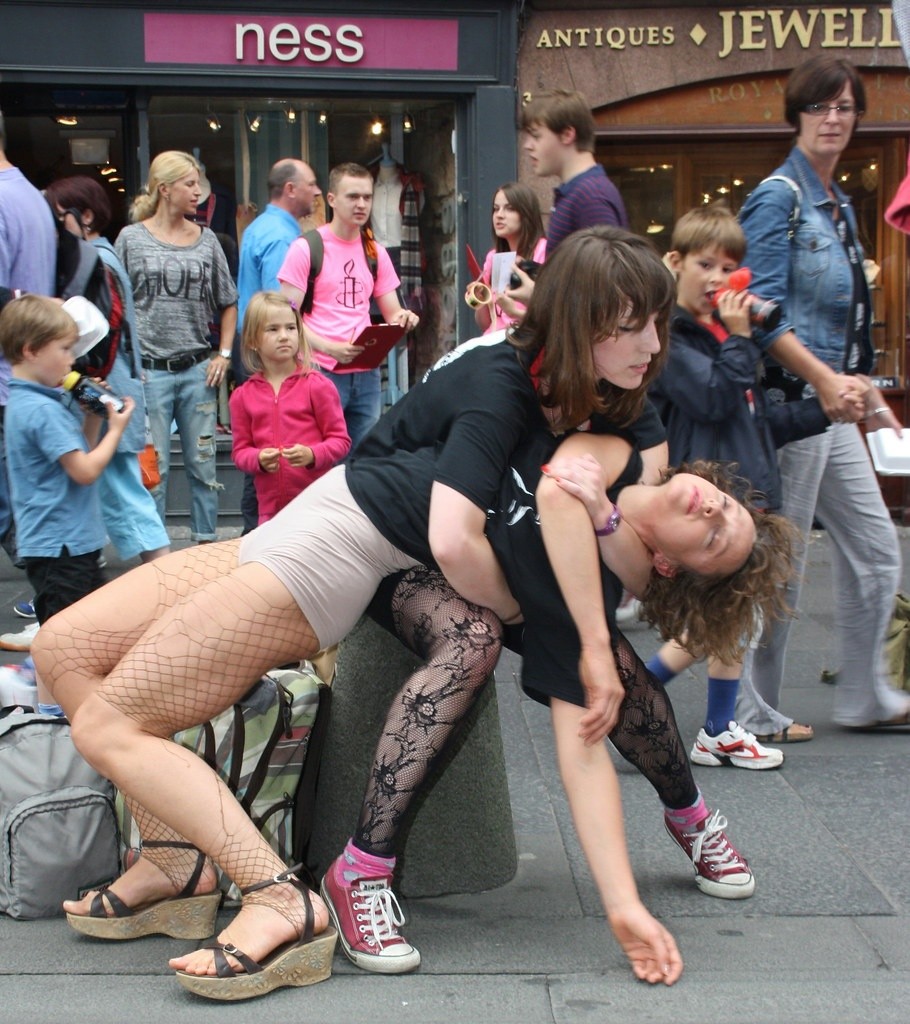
[0,704,122,918]
[49,204,133,376]
[112,659,328,904]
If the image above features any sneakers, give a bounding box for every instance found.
[0,621,40,651]
[665,809,754,898]
[321,860,420,974]
[14,599,37,618]
[690,721,785,769]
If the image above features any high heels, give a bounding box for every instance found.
[66,841,220,939]
[176,865,338,1000]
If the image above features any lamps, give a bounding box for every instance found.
[53,116,78,125]
[283,106,297,130]
[402,103,417,134]
[645,218,665,234]
[204,99,223,133]
[369,105,383,136]
[318,99,334,129]
[245,112,264,133]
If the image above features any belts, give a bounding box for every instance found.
[141,348,212,372]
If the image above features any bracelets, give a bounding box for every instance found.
[863,407,892,419]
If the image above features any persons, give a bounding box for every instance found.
[467,87,628,334]
[30,226,792,1003]
[43,175,171,561]
[231,157,419,536]
[643,58,909,770]
[0,104,60,579]
[0,297,134,720]
[362,159,431,353]
[113,151,241,545]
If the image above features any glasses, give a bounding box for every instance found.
[799,102,866,116]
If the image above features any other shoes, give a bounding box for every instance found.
[756,721,813,742]
[851,702,910,729]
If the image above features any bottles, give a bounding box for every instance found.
[63,370,123,419]
[710,288,783,331]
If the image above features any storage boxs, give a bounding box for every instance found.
[865,426,910,478]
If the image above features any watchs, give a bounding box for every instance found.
[216,348,233,359]
[594,503,623,536]
